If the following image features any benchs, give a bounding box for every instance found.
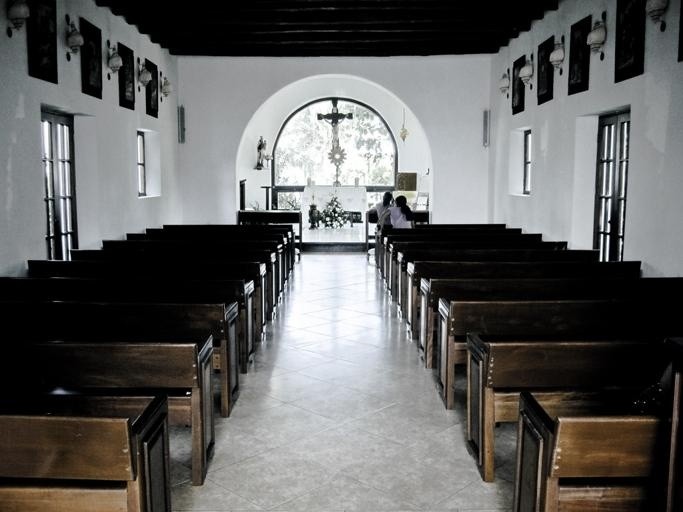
[407,261,641,340]
[437,297,683,410]
[377,228,522,272]
[0,297,239,419]
[163,225,295,270]
[389,241,567,302]
[103,240,282,305]
[382,234,542,282]
[512,392,683,512]
[1,277,254,374]
[419,276,655,369]
[0,334,216,486]
[27,259,266,343]
[70,249,277,321]
[126,233,285,292]
[466,332,683,482]
[0,395,172,512]
[396,250,599,323]
[146,228,289,281]
[375,224,506,269]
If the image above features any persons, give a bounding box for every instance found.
[373,192,395,229]
[380,196,415,229]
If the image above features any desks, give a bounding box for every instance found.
[239,209,302,261]
[366,211,430,261]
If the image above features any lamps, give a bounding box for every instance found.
[159,71,172,102]
[136,56,151,92]
[7,2,31,38]
[65,14,85,61]
[499,68,510,98]
[107,39,122,81]
[646,0,670,32]
[549,34,565,75]
[518,54,534,90]
[587,11,607,60]
